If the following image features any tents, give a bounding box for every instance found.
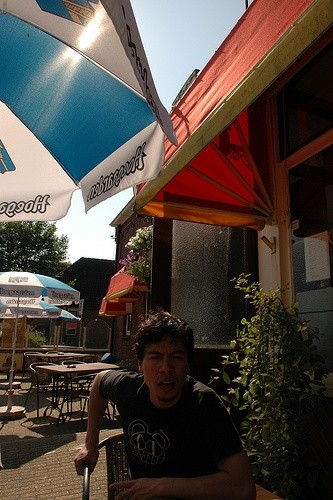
[132,0,333,314]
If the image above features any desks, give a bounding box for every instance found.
[37,362,119,415]
[27,354,91,408]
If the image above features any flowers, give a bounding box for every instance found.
[118,224,153,283]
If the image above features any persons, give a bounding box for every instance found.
[73,310,256,500]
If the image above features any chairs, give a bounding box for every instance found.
[56,373,117,426]
[23,362,70,418]
[83,433,131,500]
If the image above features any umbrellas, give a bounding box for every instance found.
[0,271,81,411]
[0,0,176,222]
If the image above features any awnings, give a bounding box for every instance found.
[98,266,149,317]
